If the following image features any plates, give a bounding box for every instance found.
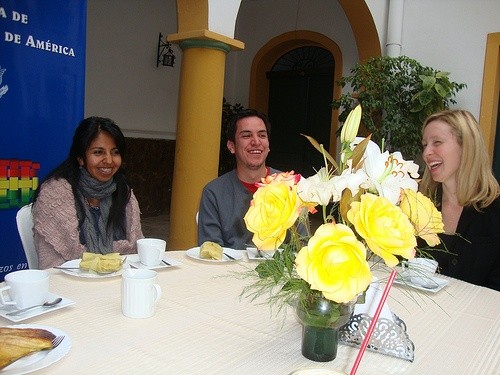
[246,247,284,260]
[61,259,132,278]
[396,275,449,293]
[130,257,180,268]
[0,324,72,375]
[186,246,245,262]
[0,281,77,322]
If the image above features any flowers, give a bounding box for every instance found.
[210,103,473,332]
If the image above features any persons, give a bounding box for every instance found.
[197,109,311,252]
[387,110,500,292]
[31,117,146,271]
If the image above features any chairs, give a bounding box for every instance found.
[15,203,38,269]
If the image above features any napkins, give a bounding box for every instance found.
[199,240,224,260]
[80,251,123,275]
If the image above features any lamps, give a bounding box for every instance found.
[156,31,177,69]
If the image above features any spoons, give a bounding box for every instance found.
[6,298,62,314]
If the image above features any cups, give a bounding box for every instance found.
[121,269,161,318]
[137,238,166,266]
[0,269,51,310]
[401,258,438,285]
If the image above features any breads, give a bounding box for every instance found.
[0,327,55,368]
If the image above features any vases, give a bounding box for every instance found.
[293,287,355,363]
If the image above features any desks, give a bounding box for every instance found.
[0,251,500,375]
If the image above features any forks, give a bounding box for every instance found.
[53,256,127,269]
[0,334,66,370]
